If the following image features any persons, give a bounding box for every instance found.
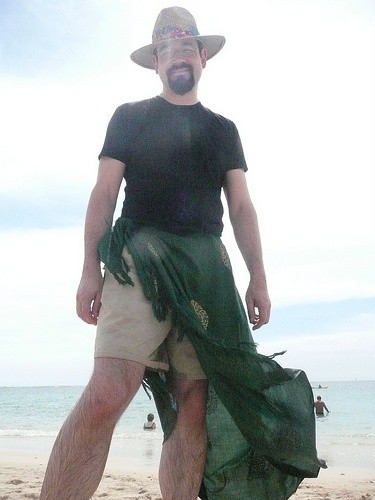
[143,414,156,430]
[314,396,330,415]
[38,8,328,500]
